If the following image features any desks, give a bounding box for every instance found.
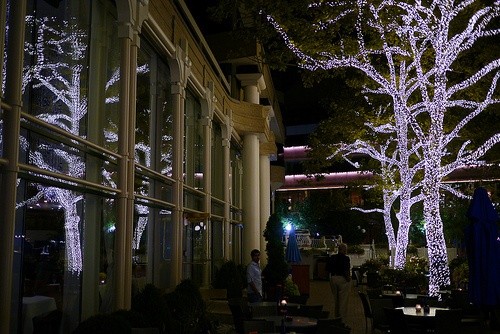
[201,258,500,334]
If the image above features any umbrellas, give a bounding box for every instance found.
[286,231,302,263]
[465,186,500,302]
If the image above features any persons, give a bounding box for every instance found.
[330,244,350,285]
[247,249,263,302]
[452,259,469,291]
